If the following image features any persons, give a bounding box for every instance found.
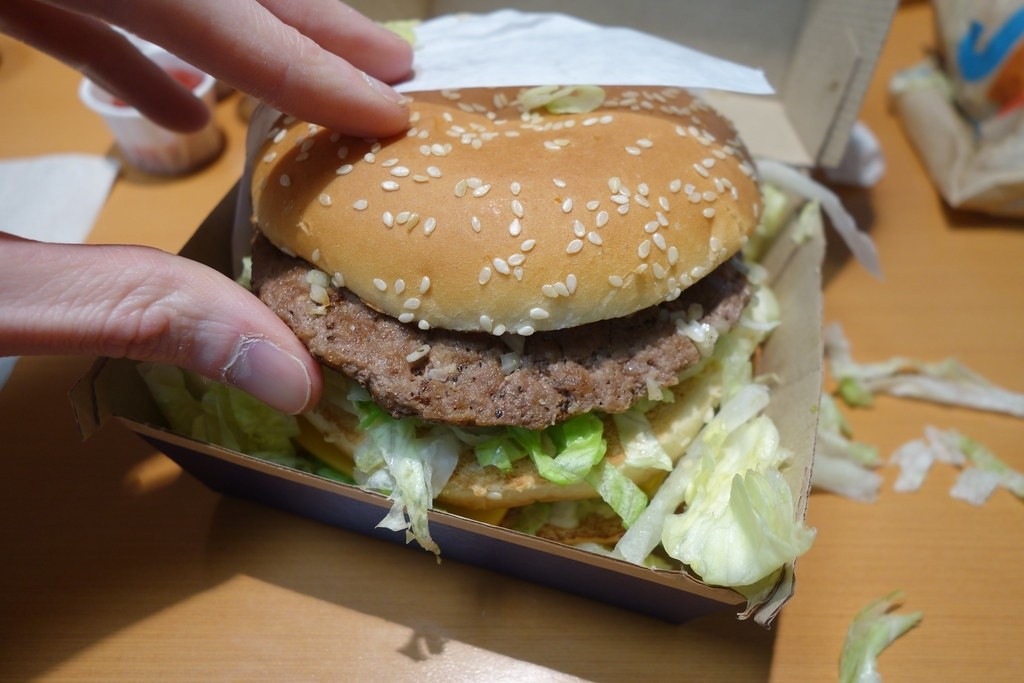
[0,0,415,417]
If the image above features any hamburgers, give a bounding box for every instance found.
[253,84,761,540]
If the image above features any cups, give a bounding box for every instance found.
[79,50,221,174]
